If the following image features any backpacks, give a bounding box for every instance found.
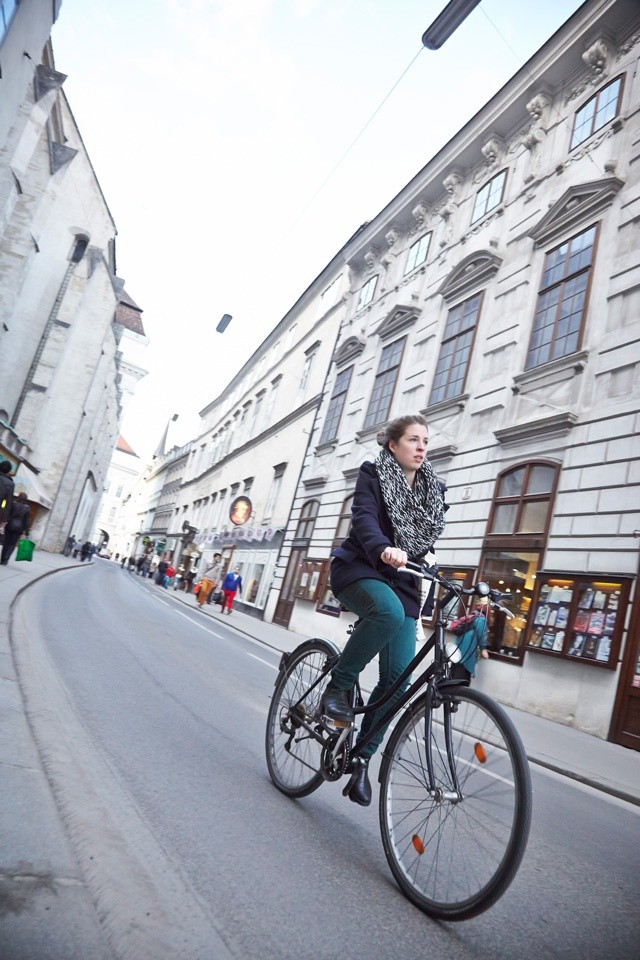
[451,613,484,636]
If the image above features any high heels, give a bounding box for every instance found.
[346,755,372,805]
[316,683,355,722]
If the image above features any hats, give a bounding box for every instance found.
[1,460,12,473]
[477,604,493,615]
[214,552,221,557]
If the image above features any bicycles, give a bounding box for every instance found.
[266,557,533,924]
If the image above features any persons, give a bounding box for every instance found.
[329,414,450,806]
[0,461,30,565]
[63,535,97,562]
[115,551,243,615]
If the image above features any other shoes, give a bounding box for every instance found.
[227,609,232,615]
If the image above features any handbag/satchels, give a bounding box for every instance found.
[193,581,201,594]
[15,537,36,562]
